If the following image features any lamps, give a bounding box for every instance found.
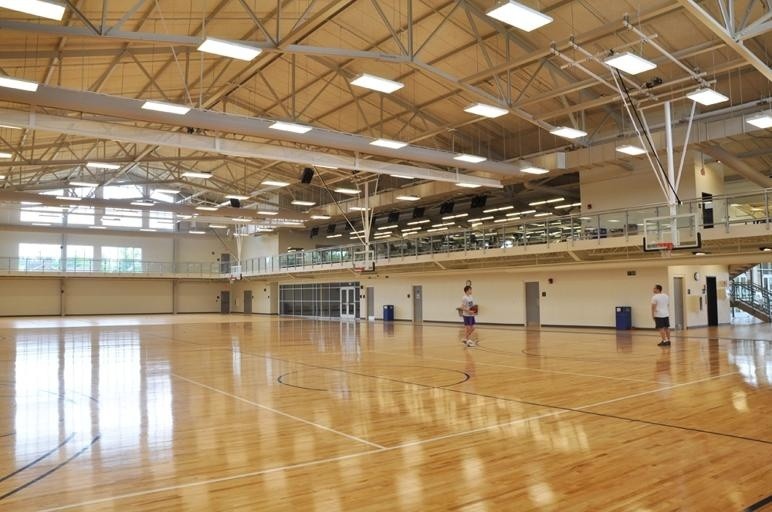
[140,19,195,117]
[350,1,405,95]
[745,77,772,128]
[517,124,550,175]
[615,109,648,156]
[483,1,554,33]
[1,1,70,23]
[603,8,658,75]
[269,51,312,134]
[0,32,42,93]
[464,19,509,120]
[196,0,263,62]
[550,84,588,139]
[687,49,729,107]
[369,90,408,150]
[450,128,489,163]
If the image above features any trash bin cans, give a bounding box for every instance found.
[616,307,631,330]
[383,305,393,320]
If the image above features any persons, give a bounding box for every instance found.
[651,284,671,346]
[459,285,479,343]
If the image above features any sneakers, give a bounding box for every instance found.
[463,338,475,347]
[657,341,670,346]
